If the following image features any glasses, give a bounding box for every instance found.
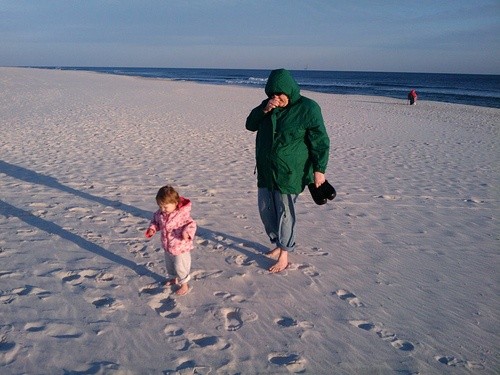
[268,91,283,96]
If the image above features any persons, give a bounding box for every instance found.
[244,68,331,273]
[145,186,198,295]
[408,89,417,105]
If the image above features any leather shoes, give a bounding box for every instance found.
[308,178,336,206]
